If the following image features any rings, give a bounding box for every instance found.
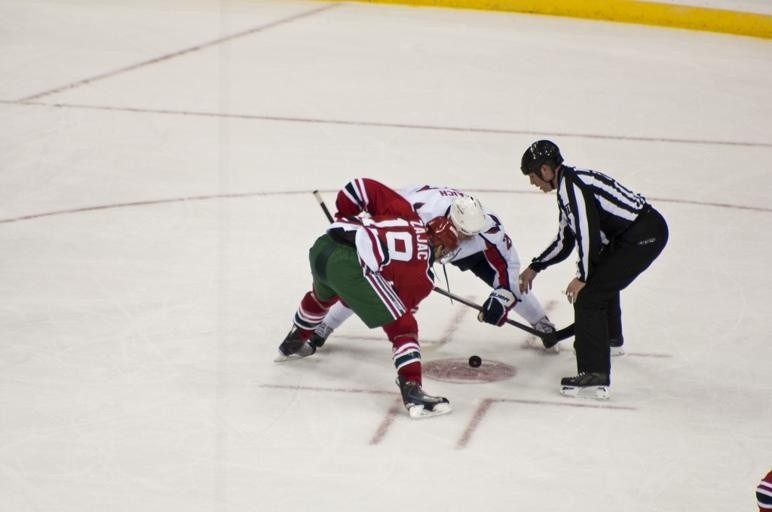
[519,279,523,284]
[570,292,573,296]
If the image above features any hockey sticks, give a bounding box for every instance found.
[430,286,576,347]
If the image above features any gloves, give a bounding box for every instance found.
[476,284,518,326]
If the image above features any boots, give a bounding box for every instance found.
[573,329,624,349]
[560,366,611,389]
[530,315,556,334]
[397,375,450,412]
[306,331,325,354]
[278,326,303,356]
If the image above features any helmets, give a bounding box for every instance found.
[426,214,460,252]
[520,139,564,176]
[449,195,485,236]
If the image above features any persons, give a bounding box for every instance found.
[298,184,557,355]
[278,178,460,410]
[518,140,669,387]
